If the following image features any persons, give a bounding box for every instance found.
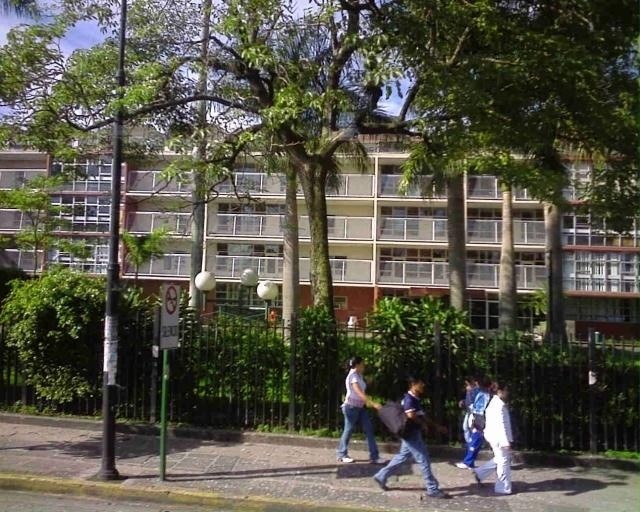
[453,376,491,470]
[336,357,386,464]
[473,381,515,495]
[372,376,448,498]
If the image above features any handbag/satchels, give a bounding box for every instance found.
[376,401,410,439]
[472,413,486,431]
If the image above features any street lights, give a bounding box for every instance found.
[240,268,258,306]
[194,270,217,314]
[256,280,278,348]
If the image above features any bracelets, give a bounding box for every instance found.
[366,400,373,407]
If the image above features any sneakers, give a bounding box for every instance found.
[372,475,389,491]
[370,458,386,464]
[336,456,354,463]
[427,489,448,498]
[456,462,472,470]
